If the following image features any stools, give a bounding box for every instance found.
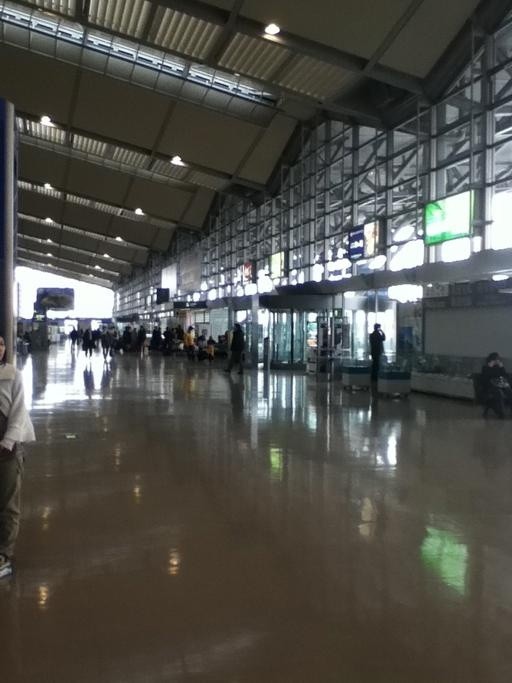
[377,371,411,399]
[342,364,371,391]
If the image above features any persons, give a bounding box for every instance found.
[222,322,245,373]
[223,372,246,424]
[99,362,111,400]
[1,331,40,578]
[69,318,218,362]
[365,382,382,444]
[83,362,96,402]
[480,348,511,418]
[368,321,387,383]
[181,366,197,404]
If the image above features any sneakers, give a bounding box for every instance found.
[0,554,13,579]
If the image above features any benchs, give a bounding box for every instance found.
[410,352,491,399]
